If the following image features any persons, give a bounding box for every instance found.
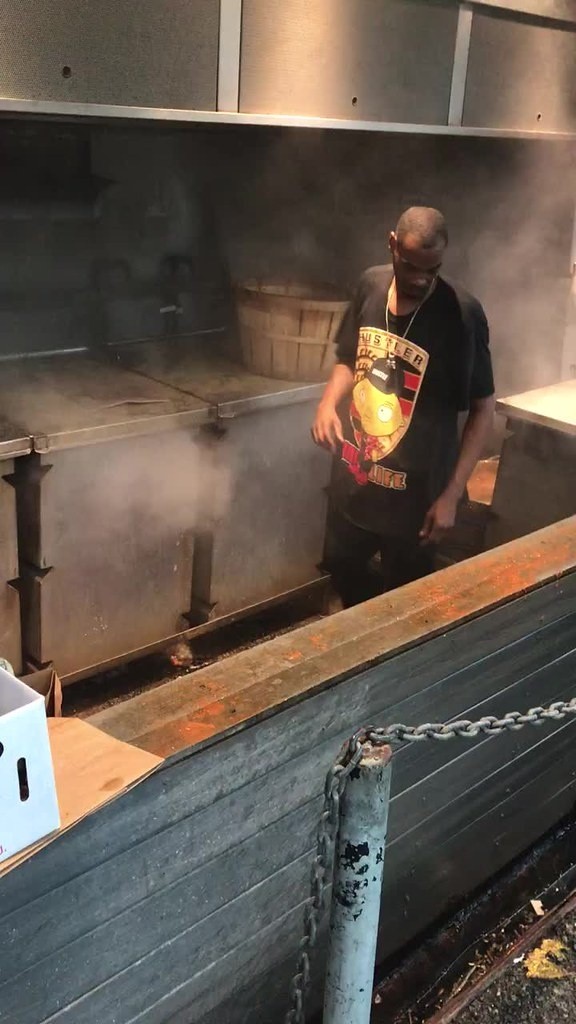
[311,206,494,608]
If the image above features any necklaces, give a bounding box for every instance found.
[385,275,436,358]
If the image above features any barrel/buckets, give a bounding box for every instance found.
[232,279,353,384]
